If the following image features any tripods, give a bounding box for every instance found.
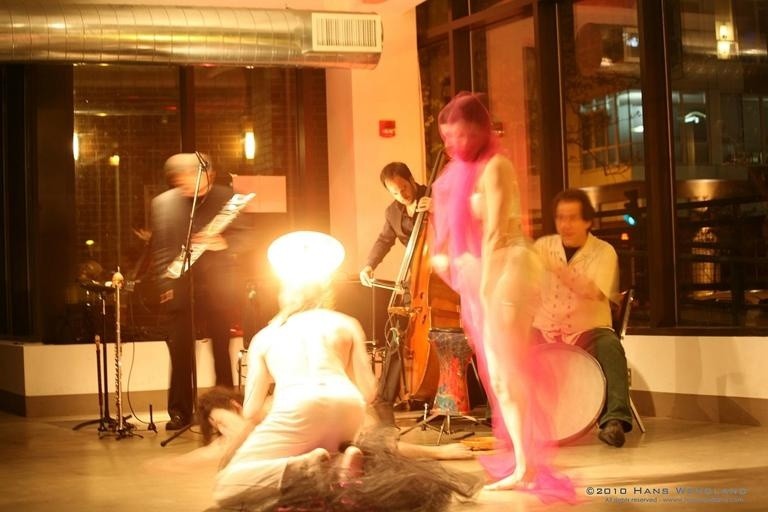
[160,262,218,448]
[98,302,144,441]
[71,318,133,438]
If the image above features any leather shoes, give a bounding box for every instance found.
[165,416,192,430]
[599,420,628,447]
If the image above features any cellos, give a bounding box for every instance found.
[377,149,461,412]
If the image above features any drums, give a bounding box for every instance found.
[538,342,607,446]
[428,326,475,418]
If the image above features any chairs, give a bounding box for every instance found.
[607,287,647,433]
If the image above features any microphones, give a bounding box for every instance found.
[195,149,208,172]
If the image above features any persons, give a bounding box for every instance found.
[145,388,476,462]
[151,153,241,431]
[533,189,632,448]
[214,284,379,503]
[425,92,543,492]
[360,162,431,412]
